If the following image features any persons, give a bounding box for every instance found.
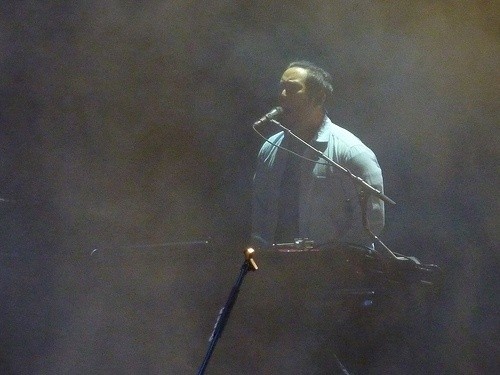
[251,61,384,252]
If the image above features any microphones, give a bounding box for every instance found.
[253,106,284,126]
